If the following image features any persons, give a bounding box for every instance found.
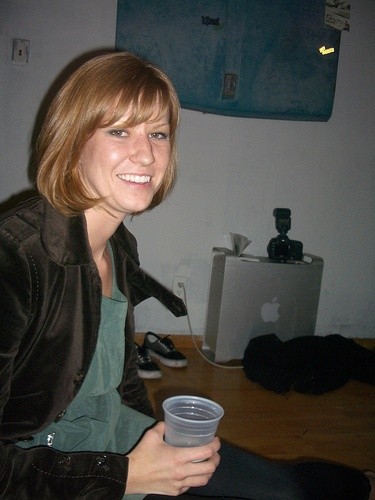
[1,48,371,499]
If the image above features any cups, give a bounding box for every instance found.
[161,395,224,448]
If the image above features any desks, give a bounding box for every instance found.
[134,332,375,473]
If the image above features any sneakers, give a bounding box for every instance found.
[136,332,189,380]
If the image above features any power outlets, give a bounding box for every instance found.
[12,38,31,65]
[171,277,188,305]
